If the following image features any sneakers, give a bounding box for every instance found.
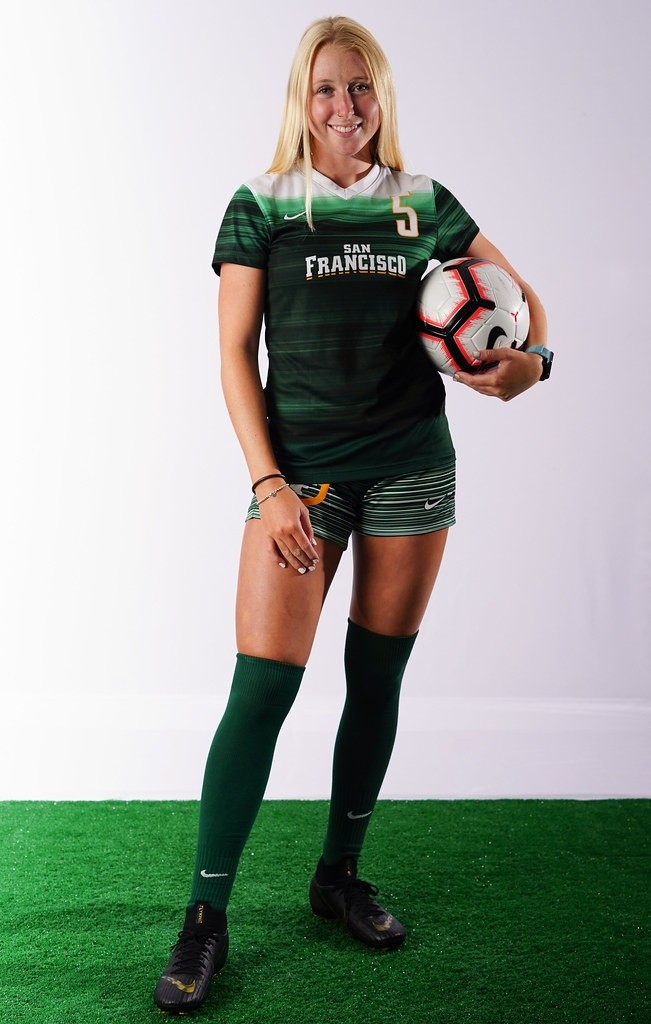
[309,855,407,951]
[152,900,229,1015]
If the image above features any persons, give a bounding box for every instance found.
[154,18,548,1011]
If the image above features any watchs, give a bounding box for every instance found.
[525,344,554,381]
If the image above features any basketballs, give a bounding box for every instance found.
[418,256,530,377]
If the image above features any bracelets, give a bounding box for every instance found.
[251,473,292,495]
[255,484,290,506]
